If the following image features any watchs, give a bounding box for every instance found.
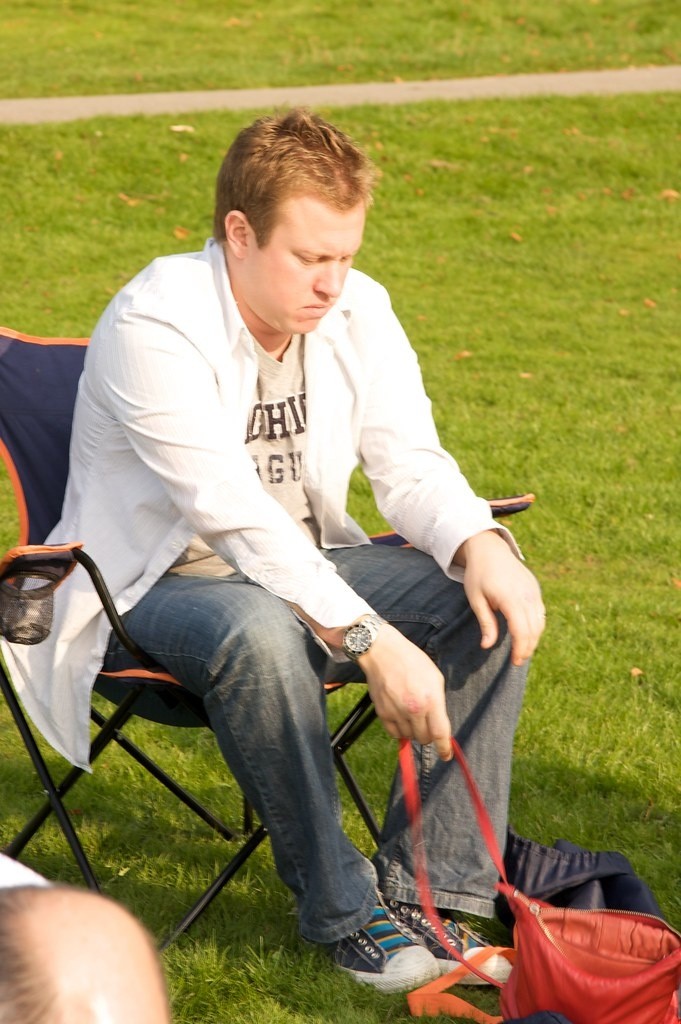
[340,616,386,662]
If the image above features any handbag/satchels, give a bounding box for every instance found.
[392,734,681,1023]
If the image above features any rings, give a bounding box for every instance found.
[539,612,546,618]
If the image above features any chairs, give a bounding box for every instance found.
[0,326,534,952]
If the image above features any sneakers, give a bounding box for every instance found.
[316,894,442,995]
[379,896,514,985]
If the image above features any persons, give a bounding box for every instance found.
[0,114,546,990]
[0,854,172,1024]
